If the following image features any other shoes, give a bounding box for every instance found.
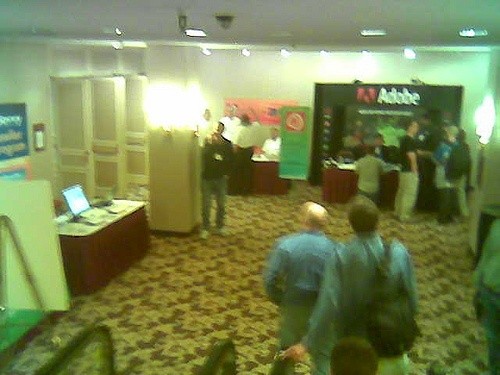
[437,216,453,223]
[200,229,208,239]
[399,213,420,223]
[219,228,231,236]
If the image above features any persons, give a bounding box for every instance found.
[280,195,419,375]
[262,127,281,155]
[264,202,336,375]
[219,105,242,144]
[433,126,469,217]
[354,144,388,206]
[200,122,234,240]
[329,336,378,375]
[391,118,431,222]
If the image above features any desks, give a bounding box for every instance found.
[58,199,151,295]
[226,159,290,195]
[320,167,399,210]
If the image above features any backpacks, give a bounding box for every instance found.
[445,142,471,176]
[360,238,422,357]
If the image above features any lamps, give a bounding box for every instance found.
[215,15,234,31]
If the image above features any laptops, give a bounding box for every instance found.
[63,183,107,225]
